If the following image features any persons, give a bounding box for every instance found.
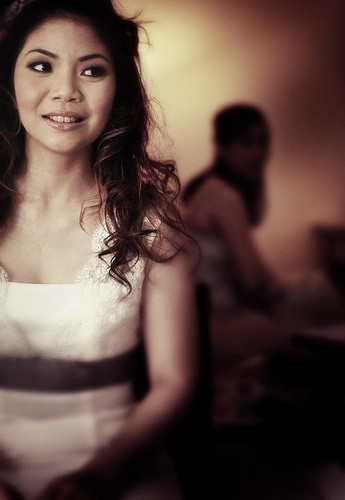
[0,0,203,500]
[175,101,298,430]
